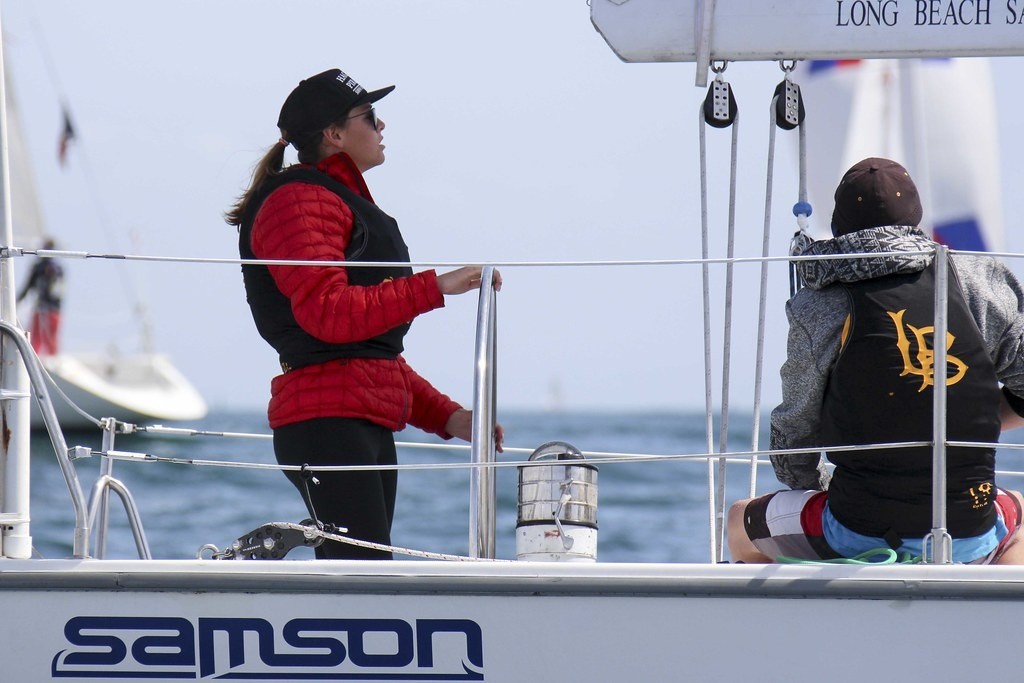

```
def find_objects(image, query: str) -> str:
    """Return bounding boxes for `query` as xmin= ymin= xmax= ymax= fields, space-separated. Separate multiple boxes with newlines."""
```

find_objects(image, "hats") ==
xmin=831 ymin=157 xmax=923 ymax=237
xmin=276 ymin=69 xmax=395 ymax=150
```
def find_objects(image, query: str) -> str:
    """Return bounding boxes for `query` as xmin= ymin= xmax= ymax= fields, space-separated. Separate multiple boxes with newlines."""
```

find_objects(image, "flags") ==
xmin=58 ymin=114 xmax=75 ymax=168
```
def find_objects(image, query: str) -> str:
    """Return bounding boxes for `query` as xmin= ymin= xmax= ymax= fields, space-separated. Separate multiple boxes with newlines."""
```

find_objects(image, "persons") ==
xmin=727 ymin=158 xmax=1024 ymax=565
xmin=222 ymin=69 xmax=504 ymax=561
xmin=14 ymin=238 xmax=65 ymax=354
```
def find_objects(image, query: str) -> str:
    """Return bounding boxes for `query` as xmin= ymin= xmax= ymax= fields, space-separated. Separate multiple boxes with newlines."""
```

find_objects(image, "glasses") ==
xmin=334 ymin=107 xmax=377 ymax=131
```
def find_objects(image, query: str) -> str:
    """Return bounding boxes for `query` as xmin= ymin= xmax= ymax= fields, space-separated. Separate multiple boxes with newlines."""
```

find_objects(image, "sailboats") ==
xmin=0 ymin=0 xmax=213 ymax=443
